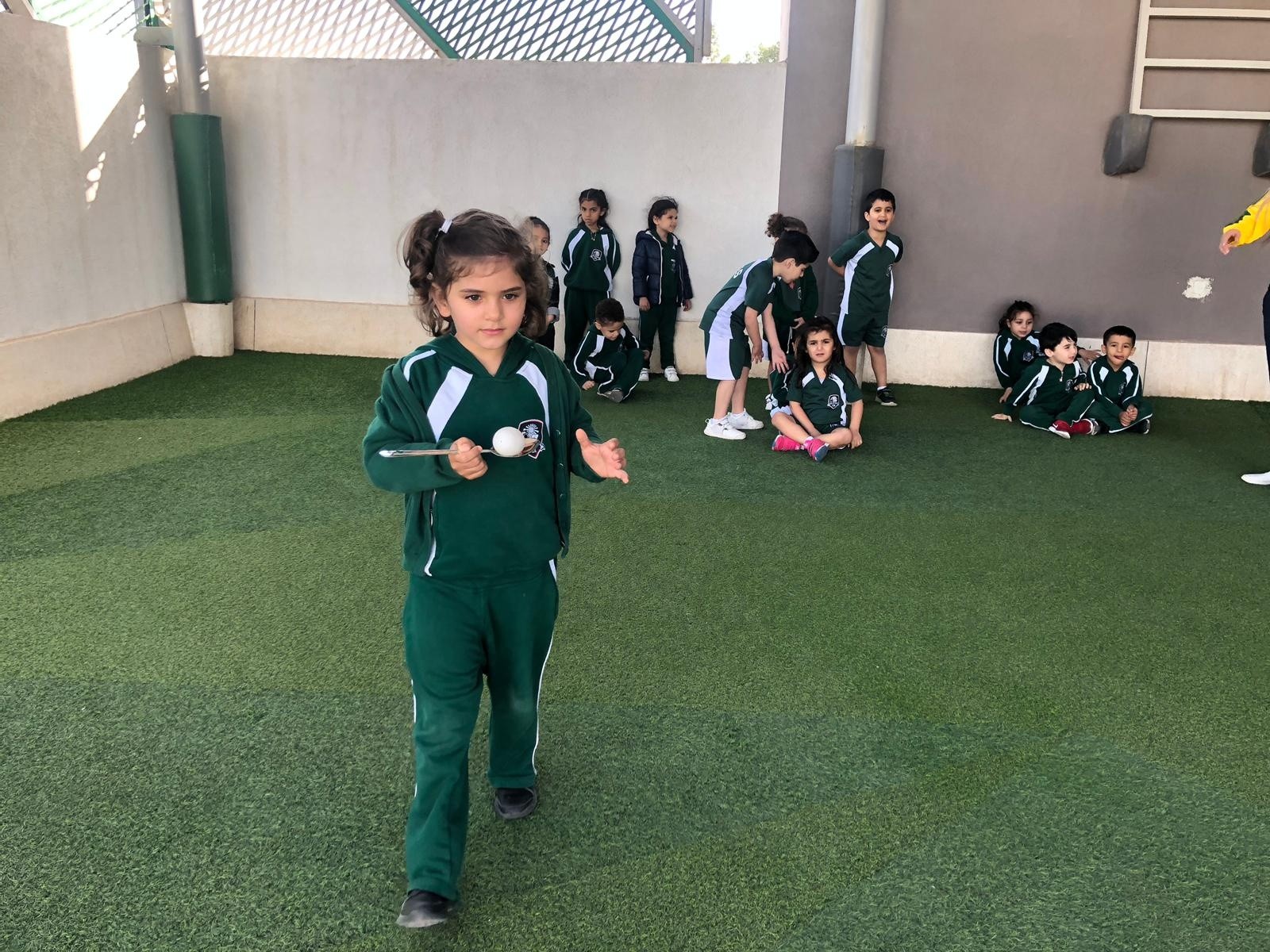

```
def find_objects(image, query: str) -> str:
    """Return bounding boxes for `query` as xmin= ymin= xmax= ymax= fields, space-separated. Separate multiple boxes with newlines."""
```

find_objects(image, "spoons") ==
xmin=379 ymin=438 xmax=541 ymax=458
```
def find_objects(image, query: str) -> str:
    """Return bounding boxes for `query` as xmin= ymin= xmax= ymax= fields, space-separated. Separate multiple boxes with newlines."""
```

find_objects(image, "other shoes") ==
xmin=596 ymin=385 xmax=624 ymax=403
xmin=1134 ymin=418 xmax=1151 ymax=435
xmin=1088 ymin=416 xmax=1104 ymax=435
xmin=1241 ymin=471 xmax=1270 ymax=485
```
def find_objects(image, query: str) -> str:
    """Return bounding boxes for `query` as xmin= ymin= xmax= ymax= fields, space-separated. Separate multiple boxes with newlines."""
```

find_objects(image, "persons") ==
xmin=574 ymin=298 xmax=643 ymax=402
xmin=828 ymin=187 xmax=905 ymax=406
xmin=362 ymin=209 xmax=627 ymax=927
xmin=632 ymin=196 xmax=694 ymax=381
xmin=766 ymin=214 xmax=819 ymax=410
xmin=991 ymin=300 xmax=1154 ymax=439
xmin=562 ymin=187 xmax=621 ymax=373
xmin=1219 ymin=187 xmax=1270 ymax=484
xmin=769 ymin=315 xmax=863 ymax=461
xmin=699 ymin=229 xmax=820 ymax=440
xmin=519 ymin=217 xmax=560 ymax=355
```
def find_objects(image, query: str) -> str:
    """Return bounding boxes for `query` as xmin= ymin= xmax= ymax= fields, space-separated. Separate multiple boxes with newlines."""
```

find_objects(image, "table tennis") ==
xmin=492 ymin=426 xmax=525 ymax=456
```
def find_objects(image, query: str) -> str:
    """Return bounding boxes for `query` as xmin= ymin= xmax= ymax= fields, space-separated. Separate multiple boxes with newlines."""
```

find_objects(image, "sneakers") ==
xmin=771 ymin=435 xmax=806 ymax=451
xmin=703 ymin=415 xmax=746 ymax=440
xmin=1048 ymin=420 xmax=1073 ymax=439
xmin=875 ymin=386 xmax=898 ymax=407
xmin=638 ymin=367 xmax=651 ymax=381
xmin=1073 ymin=418 xmax=1094 ymax=435
xmin=396 ymin=888 xmax=456 ymax=927
xmin=727 ymin=408 xmax=764 ymax=430
xmin=803 ymin=438 xmax=830 ymax=463
xmin=494 ymin=783 xmax=537 ymax=820
xmin=664 ymin=366 xmax=680 ymax=382
